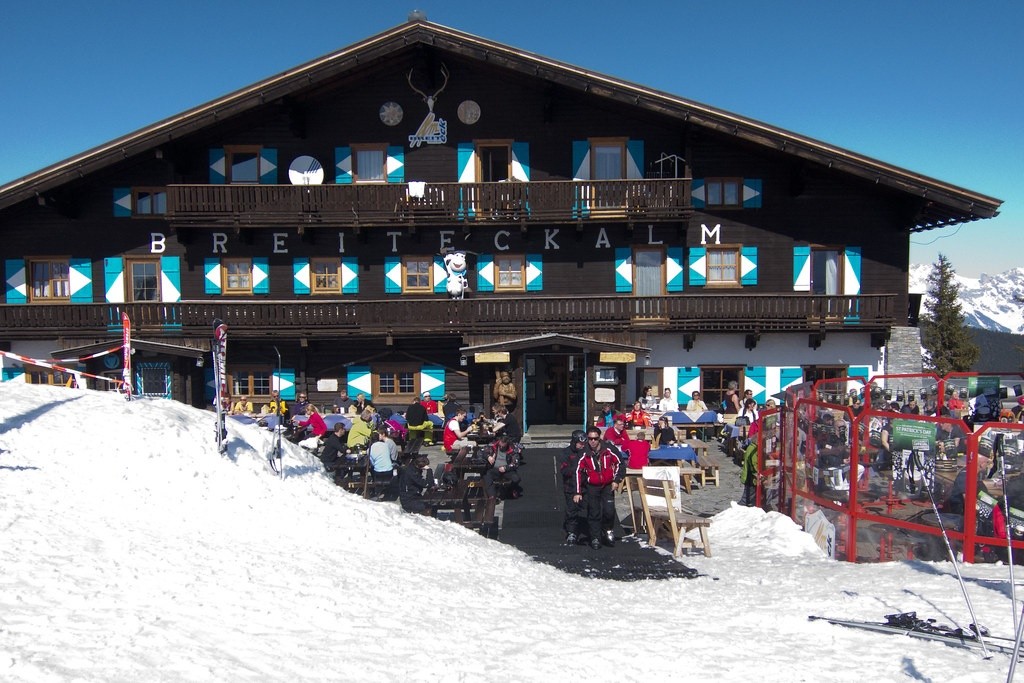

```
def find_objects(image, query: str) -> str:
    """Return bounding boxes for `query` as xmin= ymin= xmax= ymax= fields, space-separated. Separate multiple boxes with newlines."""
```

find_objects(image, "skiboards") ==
xmin=121 ymin=311 xmax=132 ymax=401
xmin=808 ymin=615 xmax=1024 ymax=657
xmin=213 ymin=318 xmax=227 ymax=442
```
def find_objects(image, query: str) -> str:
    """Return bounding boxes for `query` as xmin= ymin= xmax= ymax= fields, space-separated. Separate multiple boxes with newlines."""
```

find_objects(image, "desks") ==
xmin=621 ymin=444 xmax=697 ymax=462
xmin=933 ymin=454 xmax=966 ymax=485
xmin=373 ymin=413 xmax=444 ymax=429
xmin=424 ymin=476 xmax=474 ymax=525
xmin=332 ymin=447 xmax=367 ymax=471
xmin=467 ymin=429 xmax=495 ymax=444
xmin=228 ymin=413 xmax=279 ymax=430
xmin=452 ymin=445 xmax=493 ymax=478
xmin=293 ymin=414 xmax=384 ymax=431
xmin=662 ymin=412 xmax=718 ymax=428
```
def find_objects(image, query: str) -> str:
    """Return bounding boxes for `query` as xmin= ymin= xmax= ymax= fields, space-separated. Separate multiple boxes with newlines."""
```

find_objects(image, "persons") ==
xmin=483 ymin=436 xmax=521 ymax=499
xmin=444 ymin=408 xmax=476 ymax=451
xmin=725 ymin=381 xmax=740 ymax=414
xmin=560 ymin=430 xmax=589 ymax=544
xmin=221 ymin=392 xmax=235 ymax=414
xmin=737 ymin=389 xmax=1024 ymax=566
xmin=573 ymin=427 xmax=626 ymax=549
xmin=686 ymin=391 xmax=708 ymax=411
xmin=270 ymin=390 xmax=288 ymax=414
xmin=638 ymin=385 xmax=657 ymax=410
xmin=291 ymin=404 xmax=327 ymax=444
xmin=333 ymin=390 xmax=356 ymax=413
xmin=406 ymin=397 xmax=434 ymax=446
xmin=493 ymin=372 xmax=517 ymax=405
xmin=321 ymin=388 xmax=523 ymax=515
xmin=659 ymin=388 xmax=680 ymax=412
xmin=354 ymin=395 xmax=377 ymax=414
xmin=596 ymin=402 xmax=678 ymax=470
xmin=234 ymin=396 xmax=253 ymax=414
xmin=291 ymin=393 xmax=311 ymax=417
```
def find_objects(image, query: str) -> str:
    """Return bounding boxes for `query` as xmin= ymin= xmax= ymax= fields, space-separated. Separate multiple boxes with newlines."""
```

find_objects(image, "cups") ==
xmin=669 ymin=440 xmax=688 ymax=448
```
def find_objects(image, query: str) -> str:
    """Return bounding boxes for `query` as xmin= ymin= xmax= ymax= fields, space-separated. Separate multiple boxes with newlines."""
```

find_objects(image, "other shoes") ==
xmin=567 ymin=532 xmax=578 ymax=547
xmin=513 ymin=488 xmax=518 ymax=498
xmin=605 ymin=529 xmax=616 ymax=546
xmin=591 ymin=538 xmax=601 ymax=550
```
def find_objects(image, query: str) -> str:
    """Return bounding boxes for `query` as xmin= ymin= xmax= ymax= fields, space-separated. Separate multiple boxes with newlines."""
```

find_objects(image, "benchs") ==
xmin=637 ymin=436 xmax=719 ymax=559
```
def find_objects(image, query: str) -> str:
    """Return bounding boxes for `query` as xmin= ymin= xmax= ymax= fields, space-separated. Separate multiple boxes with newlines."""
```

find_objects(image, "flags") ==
xmin=121 ymin=312 xmax=132 ymax=392
xmin=212 ymin=318 xmax=232 ymax=408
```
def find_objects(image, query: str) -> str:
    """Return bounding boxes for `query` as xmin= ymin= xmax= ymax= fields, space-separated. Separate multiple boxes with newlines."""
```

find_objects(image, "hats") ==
xmin=978 ymin=447 xmax=990 ymax=458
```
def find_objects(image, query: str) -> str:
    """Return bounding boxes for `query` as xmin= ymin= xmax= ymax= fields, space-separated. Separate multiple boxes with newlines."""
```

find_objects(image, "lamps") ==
xmin=196 ymin=357 xmax=204 ymax=368
xmin=459 ymin=354 xmax=467 ymax=366
xmin=385 ymin=332 xmax=393 ymax=345
xmin=645 ymin=354 xmax=652 ymax=366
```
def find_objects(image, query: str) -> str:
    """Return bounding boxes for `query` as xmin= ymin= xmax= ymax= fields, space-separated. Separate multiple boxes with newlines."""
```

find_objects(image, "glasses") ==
xmin=766 ymin=404 xmax=770 ymax=406
xmin=588 ymin=437 xmax=599 ymax=440
xmin=299 ymin=396 xmax=306 ymax=398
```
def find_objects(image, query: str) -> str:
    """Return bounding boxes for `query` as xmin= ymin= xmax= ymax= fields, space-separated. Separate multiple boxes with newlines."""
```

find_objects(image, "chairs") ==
xmin=266 ymin=407 xmax=495 ymax=539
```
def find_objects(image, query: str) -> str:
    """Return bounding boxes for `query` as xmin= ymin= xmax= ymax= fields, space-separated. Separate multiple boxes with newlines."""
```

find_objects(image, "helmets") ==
xmin=572 ymin=429 xmax=588 ymax=444
